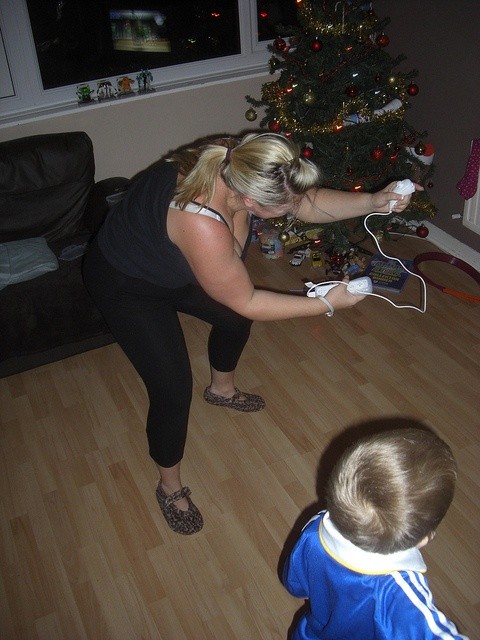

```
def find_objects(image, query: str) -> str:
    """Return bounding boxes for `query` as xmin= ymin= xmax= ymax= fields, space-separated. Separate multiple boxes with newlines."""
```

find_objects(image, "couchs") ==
xmin=1 ymin=131 xmax=131 ymax=377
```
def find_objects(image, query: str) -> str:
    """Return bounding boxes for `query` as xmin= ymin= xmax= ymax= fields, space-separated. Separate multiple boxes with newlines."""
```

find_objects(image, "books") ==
xmin=359 ymin=251 xmax=416 ymax=294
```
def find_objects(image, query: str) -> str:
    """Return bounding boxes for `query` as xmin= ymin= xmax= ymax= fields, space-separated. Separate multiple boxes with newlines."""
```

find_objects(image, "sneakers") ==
xmin=155 ymin=479 xmax=204 ymax=535
xmin=203 ymin=383 xmax=265 ymax=412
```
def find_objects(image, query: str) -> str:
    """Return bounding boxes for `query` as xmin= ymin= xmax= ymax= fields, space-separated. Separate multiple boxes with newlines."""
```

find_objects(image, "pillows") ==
xmin=0 ymin=237 xmax=58 ymax=292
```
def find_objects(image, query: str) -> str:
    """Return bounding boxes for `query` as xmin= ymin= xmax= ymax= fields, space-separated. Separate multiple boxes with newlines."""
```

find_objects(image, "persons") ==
xmin=281 ymin=425 xmax=474 ymax=639
xmin=78 ymin=128 xmax=427 ymax=536
